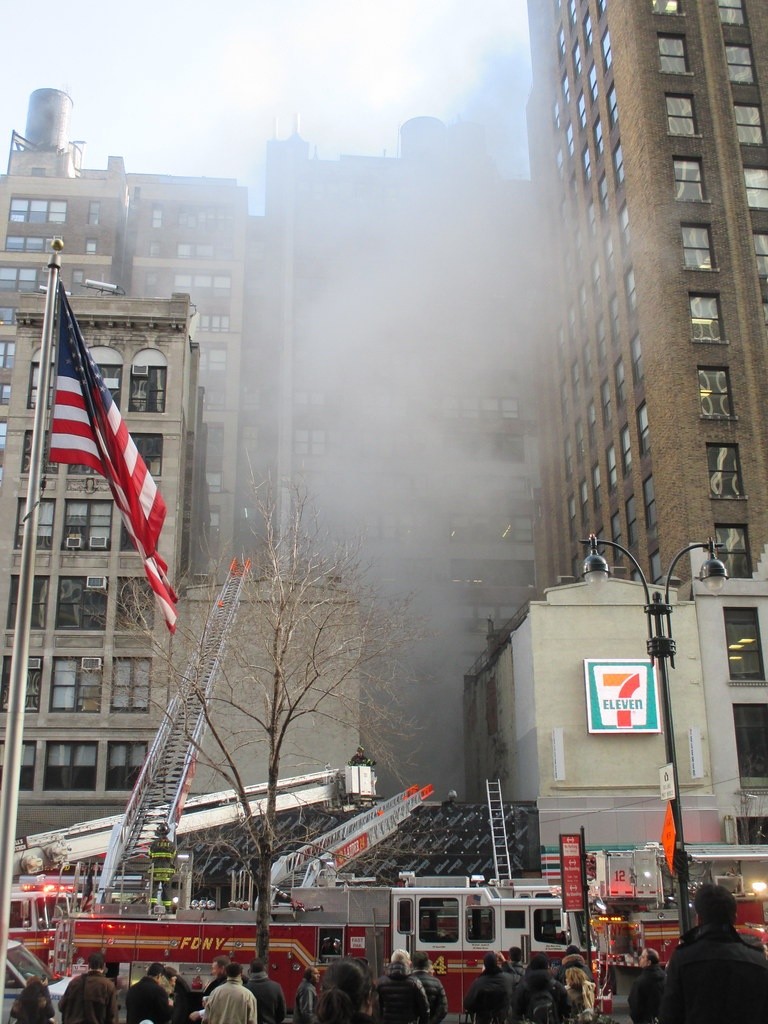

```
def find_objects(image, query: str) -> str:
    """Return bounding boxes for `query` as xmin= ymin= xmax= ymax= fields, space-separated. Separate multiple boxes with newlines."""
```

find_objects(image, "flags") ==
xmin=50 ymin=278 xmax=178 ymax=634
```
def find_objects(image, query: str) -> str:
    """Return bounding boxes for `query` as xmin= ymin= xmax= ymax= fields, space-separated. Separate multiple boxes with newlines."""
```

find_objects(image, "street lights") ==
xmin=583 ymin=532 xmax=730 ymax=936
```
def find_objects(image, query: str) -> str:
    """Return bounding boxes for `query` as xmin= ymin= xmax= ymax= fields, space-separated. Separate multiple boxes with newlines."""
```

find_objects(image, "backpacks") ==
xmin=523 ymin=979 xmax=560 ymax=1024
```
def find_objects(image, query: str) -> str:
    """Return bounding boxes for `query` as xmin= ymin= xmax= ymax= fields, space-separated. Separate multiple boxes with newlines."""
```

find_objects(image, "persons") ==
xmin=11 ymin=975 xmax=55 ymax=1024
xmin=662 ymin=884 xmax=768 ymax=1024
xmin=58 ymin=954 xmax=119 ymax=1024
xmin=126 ymin=946 xmax=594 ymax=1024
xmin=627 ymin=947 xmax=664 ymax=1024
xmin=349 ymin=747 xmax=376 ymax=767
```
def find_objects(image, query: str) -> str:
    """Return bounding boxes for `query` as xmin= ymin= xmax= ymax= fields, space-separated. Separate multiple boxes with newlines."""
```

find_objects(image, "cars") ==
xmin=4 ymin=939 xmax=71 ymax=1024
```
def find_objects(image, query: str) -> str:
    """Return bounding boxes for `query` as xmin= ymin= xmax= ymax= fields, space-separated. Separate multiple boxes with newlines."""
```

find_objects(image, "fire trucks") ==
xmin=583 ymin=844 xmax=768 ymax=959
xmin=68 ymin=556 xmax=613 ymax=1023
xmin=254 ymin=785 xmax=588 ymax=936
xmin=7 ymin=765 xmax=388 ymax=981
